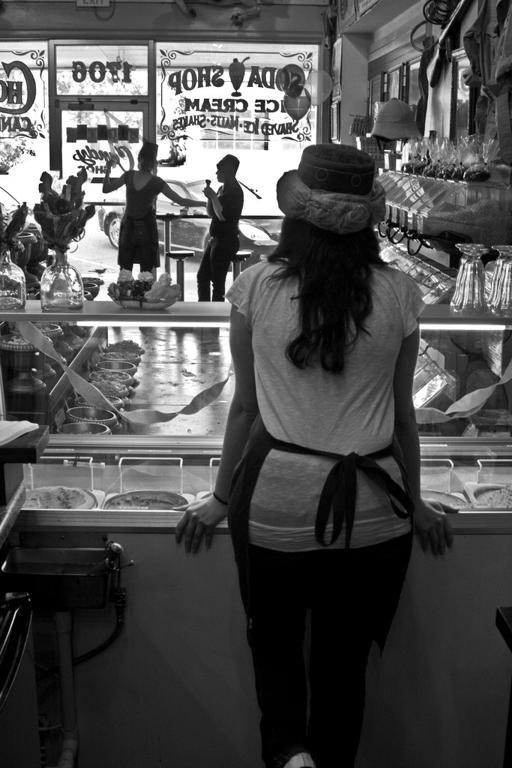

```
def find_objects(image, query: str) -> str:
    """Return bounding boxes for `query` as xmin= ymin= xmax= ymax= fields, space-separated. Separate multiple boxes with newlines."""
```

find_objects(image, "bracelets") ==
xmin=212 ymin=490 xmax=230 ymax=507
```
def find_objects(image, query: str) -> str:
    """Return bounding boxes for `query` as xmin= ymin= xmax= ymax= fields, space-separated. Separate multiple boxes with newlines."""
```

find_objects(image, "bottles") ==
xmin=0 ymin=248 xmax=29 ymax=313
xmin=37 ymin=246 xmax=88 ymax=312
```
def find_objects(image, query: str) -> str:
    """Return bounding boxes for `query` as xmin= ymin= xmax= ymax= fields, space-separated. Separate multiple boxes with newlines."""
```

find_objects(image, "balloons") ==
xmin=283 ymin=87 xmax=311 ymax=122
xmin=304 ymin=69 xmax=333 ymax=106
xmin=280 ymin=62 xmax=305 ymax=100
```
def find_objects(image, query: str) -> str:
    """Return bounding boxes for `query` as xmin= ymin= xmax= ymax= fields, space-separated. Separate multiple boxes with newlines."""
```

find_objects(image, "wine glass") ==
xmin=449 ymin=241 xmax=511 ymax=318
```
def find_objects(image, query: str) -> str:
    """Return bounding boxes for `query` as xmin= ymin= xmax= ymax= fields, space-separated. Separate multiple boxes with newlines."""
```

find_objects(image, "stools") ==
xmin=167 ymin=250 xmax=196 ymax=301
xmin=231 ymin=250 xmax=252 ymax=282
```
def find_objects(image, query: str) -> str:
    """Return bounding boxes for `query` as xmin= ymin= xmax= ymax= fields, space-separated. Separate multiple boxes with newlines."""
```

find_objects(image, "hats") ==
xmin=372 ymin=95 xmax=423 ymax=141
xmin=216 ymin=153 xmax=239 ymax=175
xmin=274 ymin=139 xmax=390 ymax=234
xmin=138 ymin=141 xmax=158 ymax=160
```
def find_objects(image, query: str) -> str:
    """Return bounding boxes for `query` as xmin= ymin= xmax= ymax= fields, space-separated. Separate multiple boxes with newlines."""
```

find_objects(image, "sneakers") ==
xmin=282 ymin=751 xmax=316 ymax=767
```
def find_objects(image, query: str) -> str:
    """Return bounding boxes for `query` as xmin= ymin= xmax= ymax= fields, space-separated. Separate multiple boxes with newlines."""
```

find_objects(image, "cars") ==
xmin=98 ymin=173 xmax=279 ymax=272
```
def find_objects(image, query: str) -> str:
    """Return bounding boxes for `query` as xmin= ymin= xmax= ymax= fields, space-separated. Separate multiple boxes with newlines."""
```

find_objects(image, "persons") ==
xmin=198 ymin=154 xmax=244 ymax=302
xmin=177 ymin=142 xmax=455 ymax=766
xmin=103 ymin=142 xmax=207 ymax=281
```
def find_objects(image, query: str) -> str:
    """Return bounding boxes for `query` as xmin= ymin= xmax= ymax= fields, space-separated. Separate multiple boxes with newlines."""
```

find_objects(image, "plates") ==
xmin=107 ymin=292 xmax=176 ymax=311
